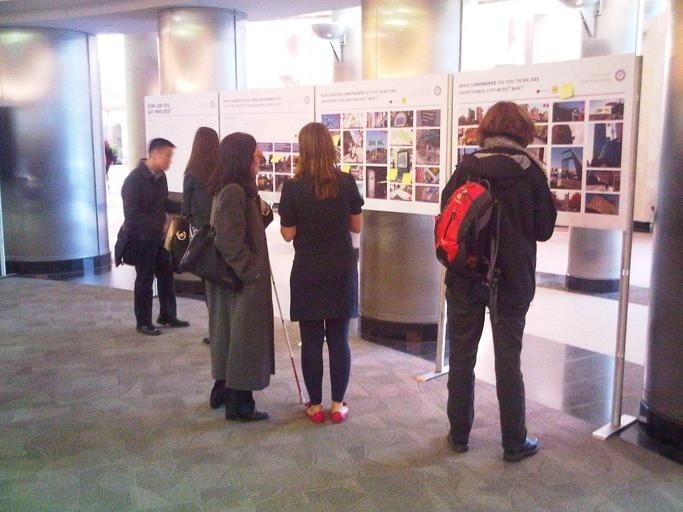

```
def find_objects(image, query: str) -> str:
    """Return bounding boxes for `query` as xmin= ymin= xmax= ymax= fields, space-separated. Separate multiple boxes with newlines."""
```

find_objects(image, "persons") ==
xmin=207 ymin=131 xmax=276 ymax=422
xmin=440 ymin=101 xmax=558 ymax=463
xmin=120 ymin=137 xmax=190 ymax=334
xmin=278 ymin=122 xmax=363 ymax=424
xmin=180 ymin=127 xmax=221 ymax=344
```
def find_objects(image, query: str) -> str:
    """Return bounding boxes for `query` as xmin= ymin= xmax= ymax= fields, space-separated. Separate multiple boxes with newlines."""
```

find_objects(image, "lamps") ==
xmin=313 ymin=21 xmax=346 ymax=60
xmin=561 ymin=0 xmax=599 ymax=37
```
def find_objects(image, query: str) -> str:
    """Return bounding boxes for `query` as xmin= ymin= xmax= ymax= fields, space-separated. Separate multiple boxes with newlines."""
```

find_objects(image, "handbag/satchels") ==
xmin=164 ymin=220 xmax=178 ymax=252
xmin=115 ymin=226 xmax=145 ymax=266
xmin=179 ymin=227 xmax=242 ymax=289
xmin=171 ymin=218 xmax=189 ymax=263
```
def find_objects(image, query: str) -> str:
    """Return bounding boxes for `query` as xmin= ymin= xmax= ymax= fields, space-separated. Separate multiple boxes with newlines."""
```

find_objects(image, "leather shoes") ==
xmin=136 ymin=324 xmax=161 ymax=335
xmin=157 ymin=318 xmax=189 ymax=327
xmin=304 ymin=401 xmax=325 ymax=422
xmin=331 ymin=401 xmax=349 ymax=423
xmin=447 ymin=432 xmax=469 ymax=452
xmin=226 ymin=408 xmax=267 ymax=420
xmin=503 ymin=438 xmax=538 ymax=462
xmin=210 ymin=388 xmax=225 ymax=409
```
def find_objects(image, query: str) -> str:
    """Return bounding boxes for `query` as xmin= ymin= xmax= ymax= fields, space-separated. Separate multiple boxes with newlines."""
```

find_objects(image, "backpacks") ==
xmin=434 ymin=171 xmax=502 ymax=282
